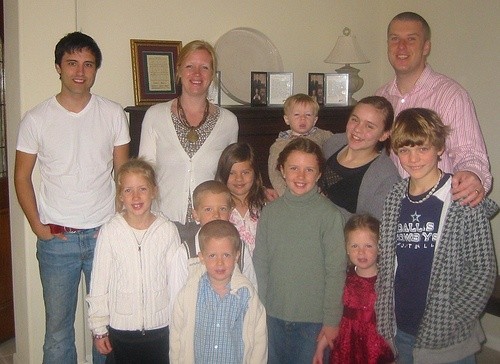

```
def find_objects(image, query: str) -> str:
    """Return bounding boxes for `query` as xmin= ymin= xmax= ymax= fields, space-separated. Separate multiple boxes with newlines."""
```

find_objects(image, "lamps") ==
xmin=323 ymin=25 xmax=370 ymax=105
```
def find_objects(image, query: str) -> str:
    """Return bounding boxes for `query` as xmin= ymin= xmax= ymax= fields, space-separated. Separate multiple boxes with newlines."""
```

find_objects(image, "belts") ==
xmin=49 ymin=224 xmax=79 ymax=234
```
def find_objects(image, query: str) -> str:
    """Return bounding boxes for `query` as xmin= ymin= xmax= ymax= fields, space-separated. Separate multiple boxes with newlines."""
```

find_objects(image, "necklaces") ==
xmin=404 ymin=168 xmax=442 ymax=204
xmin=176 ymin=95 xmax=210 ymax=143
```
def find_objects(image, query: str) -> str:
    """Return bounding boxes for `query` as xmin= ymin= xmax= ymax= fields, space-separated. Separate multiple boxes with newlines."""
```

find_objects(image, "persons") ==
xmin=375 ymin=11 xmax=493 ymax=207
xmin=268 ymin=92 xmax=334 ymax=196
xmin=372 ymin=104 xmax=500 ymax=364
xmin=311 ymin=75 xmax=323 ymax=103
xmin=312 ymin=214 xmax=397 ymax=364
xmin=167 ymin=142 xmax=267 ymax=364
xmin=317 ymin=95 xmax=401 ymax=225
xmin=87 ymin=158 xmax=183 ymax=364
xmin=13 ymin=32 xmax=131 ymax=364
xmin=140 ymin=39 xmax=280 ymax=238
xmin=252 ymin=74 xmax=267 ymax=106
xmin=254 ymin=139 xmax=345 ymax=364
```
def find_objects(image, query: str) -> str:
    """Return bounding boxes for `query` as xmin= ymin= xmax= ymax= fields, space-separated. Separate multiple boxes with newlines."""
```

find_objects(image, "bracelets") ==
xmin=92 ymin=332 xmax=109 ymax=340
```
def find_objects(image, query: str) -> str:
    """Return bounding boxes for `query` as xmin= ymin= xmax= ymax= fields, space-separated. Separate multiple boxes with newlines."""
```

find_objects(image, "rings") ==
xmin=475 ymin=190 xmax=480 ymax=195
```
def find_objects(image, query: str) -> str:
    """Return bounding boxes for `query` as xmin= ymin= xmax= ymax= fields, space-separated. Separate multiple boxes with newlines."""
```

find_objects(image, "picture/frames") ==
xmin=324 ymin=73 xmax=350 ymax=108
xmin=206 ymin=70 xmax=222 ymax=107
xmin=251 ymin=71 xmax=267 ymax=107
xmin=308 ymin=73 xmax=324 ymax=105
xmin=266 ymin=72 xmax=294 ymax=108
